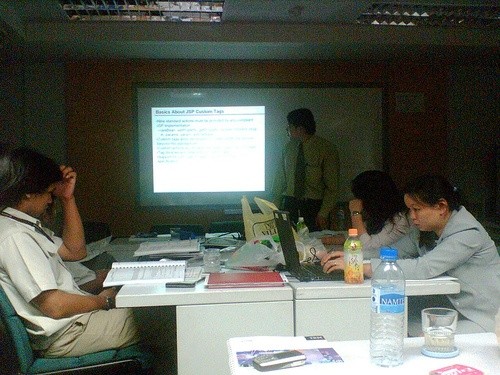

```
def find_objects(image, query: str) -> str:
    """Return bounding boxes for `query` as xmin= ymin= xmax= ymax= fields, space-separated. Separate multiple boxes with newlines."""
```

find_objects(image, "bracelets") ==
xmin=351 ymin=211 xmax=362 ymax=215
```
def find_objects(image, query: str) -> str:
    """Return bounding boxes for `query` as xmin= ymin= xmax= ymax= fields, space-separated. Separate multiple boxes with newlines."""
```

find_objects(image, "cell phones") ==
xmin=252 ymin=350 xmax=308 ymax=373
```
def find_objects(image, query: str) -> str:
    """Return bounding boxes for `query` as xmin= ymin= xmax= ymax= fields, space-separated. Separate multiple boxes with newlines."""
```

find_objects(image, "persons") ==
xmin=320 ymin=177 xmax=500 ymax=337
xmin=320 ymin=171 xmax=419 ymax=259
xmin=271 ymin=109 xmax=340 ymax=231
xmin=1 ymin=148 xmax=176 ymax=374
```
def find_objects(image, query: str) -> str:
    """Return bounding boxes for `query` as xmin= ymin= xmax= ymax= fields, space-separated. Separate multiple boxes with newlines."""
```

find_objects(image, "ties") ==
xmin=295 ymin=143 xmax=305 ymax=201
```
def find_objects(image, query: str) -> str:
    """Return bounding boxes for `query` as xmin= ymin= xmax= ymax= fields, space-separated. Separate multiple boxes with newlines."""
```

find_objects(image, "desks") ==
xmin=229 ymin=331 xmax=500 ymax=372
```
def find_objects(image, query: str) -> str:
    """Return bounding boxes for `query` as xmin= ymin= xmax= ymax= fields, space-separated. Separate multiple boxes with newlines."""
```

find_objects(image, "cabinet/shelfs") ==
xmin=286 ymin=230 xmax=460 ymax=338
xmin=118 ymin=240 xmax=293 ymax=372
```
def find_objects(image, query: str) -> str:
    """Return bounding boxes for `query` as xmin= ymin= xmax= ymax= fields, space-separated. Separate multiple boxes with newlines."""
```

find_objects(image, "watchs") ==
xmin=99 ymin=293 xmax=113 ymax=308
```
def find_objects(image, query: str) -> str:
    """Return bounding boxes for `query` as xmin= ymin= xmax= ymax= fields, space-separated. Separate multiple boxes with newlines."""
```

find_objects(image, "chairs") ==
xmin=0 ymin=289 xmax=153 ymax=374
xmin=63 ymin=222 xmax=111 ymax=244
xmin=90 ymin=239 xmax=97 ymax=243
xmin=154 ymin=221 xmax=209 ymax=240
xmin=213 ymin=216 xmax=260 ymax=239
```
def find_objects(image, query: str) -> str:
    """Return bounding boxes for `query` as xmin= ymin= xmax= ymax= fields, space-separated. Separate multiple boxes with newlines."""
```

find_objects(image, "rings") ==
xmin=335 ymin=260 xmax=336 ymax=265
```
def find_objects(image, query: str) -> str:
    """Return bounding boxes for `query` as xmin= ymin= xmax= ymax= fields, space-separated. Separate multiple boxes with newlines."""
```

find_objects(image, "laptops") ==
xmin=272 ymin=210 xmax=370 ymax=283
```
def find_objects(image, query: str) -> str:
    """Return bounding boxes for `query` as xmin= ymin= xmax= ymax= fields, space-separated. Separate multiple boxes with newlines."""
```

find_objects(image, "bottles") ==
xmin=343 ymin=229 xmax=364 ymax=284
xmin=369 ymin=247 xmax=405 ymax=356
xmin=296 ymin=217 xmax=309 ymax=237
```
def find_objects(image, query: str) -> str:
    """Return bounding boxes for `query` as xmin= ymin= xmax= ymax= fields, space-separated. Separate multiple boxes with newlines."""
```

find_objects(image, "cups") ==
xmin=420 ymin=307 xmax=459 ymax=357
xmin=202 ymin=249 xmax=222 ymax=273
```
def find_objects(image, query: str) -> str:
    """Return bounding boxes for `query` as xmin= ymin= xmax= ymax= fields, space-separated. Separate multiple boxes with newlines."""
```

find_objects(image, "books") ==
xmin=102 ymin=260 xmax=187 ymax=287
xmin=204 ymin=272 xmax=289 ymax=289
xmin=134 ymin=239 xmax=204 ymax=258
